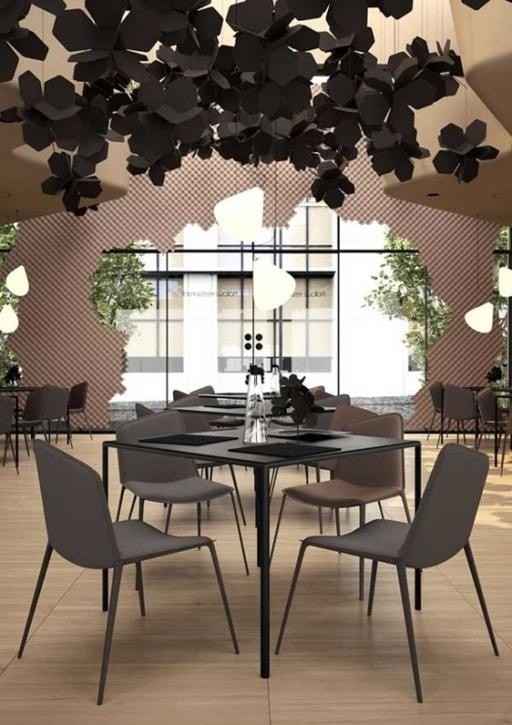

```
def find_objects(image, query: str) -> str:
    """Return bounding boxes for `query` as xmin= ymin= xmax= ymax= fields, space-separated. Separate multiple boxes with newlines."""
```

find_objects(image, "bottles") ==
xmin=242 ymin=362 xmax=269 ymax=445
xmin=269 ymin=364 xmax=282 ymax=396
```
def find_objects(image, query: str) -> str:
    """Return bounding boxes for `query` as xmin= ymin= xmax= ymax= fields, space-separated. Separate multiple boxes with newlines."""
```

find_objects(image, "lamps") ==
xmin=210 ymin=184 xmax=297 ymax=315
xmin=463 ymin=261 xmax=512 ymax=336
xmin=1 ymin=261 xmax=32 ymax=336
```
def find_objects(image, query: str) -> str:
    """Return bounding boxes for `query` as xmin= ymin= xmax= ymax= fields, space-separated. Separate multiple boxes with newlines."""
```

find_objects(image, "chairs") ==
xmin=103 ymin=385 xmax=423 ymax=679
xmin=19 ymin=439 xmax=240 ymax=705
xmin=1 ymin=381 xmax=92 ymax=475
xmin=427 ymin=381 xmax=512 ymax=477
xmin=275 ymin=442 xmax=499 ymax=703
